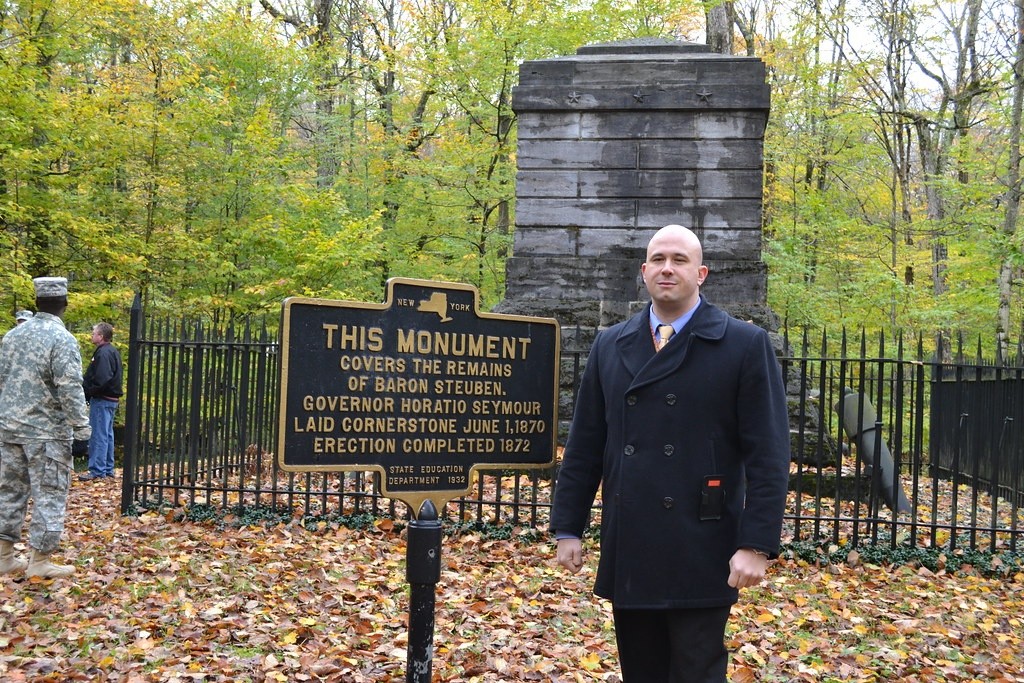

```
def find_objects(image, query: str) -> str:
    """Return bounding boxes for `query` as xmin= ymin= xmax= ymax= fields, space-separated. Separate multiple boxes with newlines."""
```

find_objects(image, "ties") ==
xmin=658 ymin=326 xmax=674 ymax=350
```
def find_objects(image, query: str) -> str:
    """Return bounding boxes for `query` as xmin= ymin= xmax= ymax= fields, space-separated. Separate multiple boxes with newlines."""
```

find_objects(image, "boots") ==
xmin=27 ymin=546 xmax=76 ymax=579
xmin=0 ymin=540 xmax=28 ymax=575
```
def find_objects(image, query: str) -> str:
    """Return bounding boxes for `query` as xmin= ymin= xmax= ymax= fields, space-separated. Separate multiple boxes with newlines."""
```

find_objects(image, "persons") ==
xmin=1 ymin=276 xmax=93 ymax=577
xmin=547 ymin=225 xmax=790 ymax=682
xmin=15 ymin=309 xmax=33 ymax=326
xmin=78 ymin=321 xmax=124 ymax=481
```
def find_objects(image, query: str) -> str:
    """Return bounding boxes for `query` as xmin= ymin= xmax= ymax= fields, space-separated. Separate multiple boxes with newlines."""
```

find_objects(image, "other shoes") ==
xmin=79 ymin=473 xmax=105 ymax=481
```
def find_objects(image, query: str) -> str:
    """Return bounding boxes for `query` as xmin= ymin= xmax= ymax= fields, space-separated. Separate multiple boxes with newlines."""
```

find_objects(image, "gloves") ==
xmin=71 ymin=439 xmax=89 ymax=458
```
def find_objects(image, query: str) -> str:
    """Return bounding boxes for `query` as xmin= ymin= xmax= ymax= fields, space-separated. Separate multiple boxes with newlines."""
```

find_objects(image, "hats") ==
xmin=15 ymin=310 xmax=33 ymax=321
xmin=33 ymin=277 xmax=68 ymax=297
